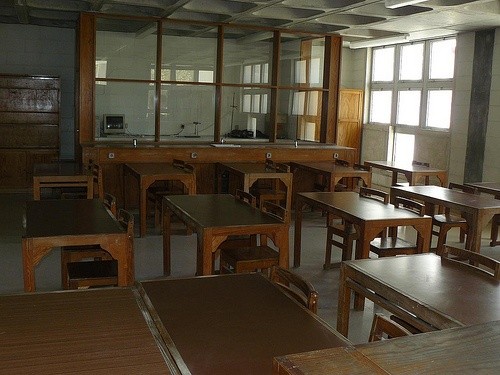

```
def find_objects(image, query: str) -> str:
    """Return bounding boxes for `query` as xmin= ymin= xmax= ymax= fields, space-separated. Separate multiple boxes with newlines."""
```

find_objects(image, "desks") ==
xmin=1 ymin=160 xmax=499 ymax=375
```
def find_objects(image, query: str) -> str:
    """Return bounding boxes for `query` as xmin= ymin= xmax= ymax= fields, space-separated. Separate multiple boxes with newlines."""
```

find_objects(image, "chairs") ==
xmin=56 ymin=158 xmax=500 ymax=341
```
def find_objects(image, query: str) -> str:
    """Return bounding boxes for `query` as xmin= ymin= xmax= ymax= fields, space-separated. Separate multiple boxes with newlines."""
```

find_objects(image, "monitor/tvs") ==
xmin=103 ymin=114 xmax=126 ymax=135
